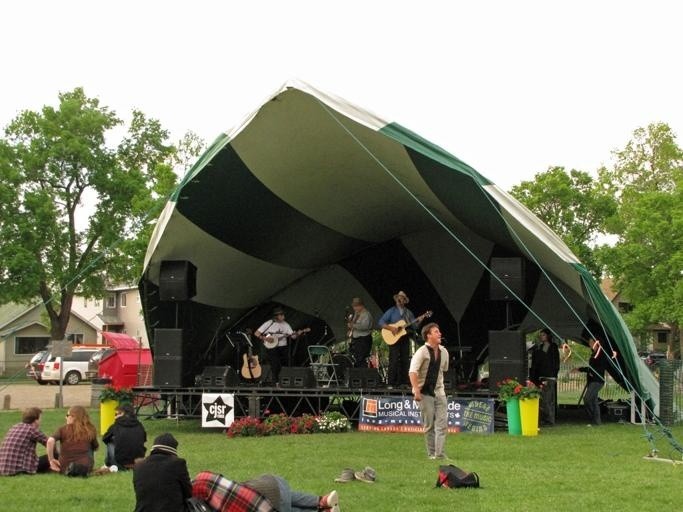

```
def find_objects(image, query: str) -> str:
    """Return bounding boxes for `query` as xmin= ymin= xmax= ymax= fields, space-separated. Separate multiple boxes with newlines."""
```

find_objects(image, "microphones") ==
xmin=324 ymin=325 xmax=328 ymax=335
xmin=220 ymin=315 xmax=231 ymax=320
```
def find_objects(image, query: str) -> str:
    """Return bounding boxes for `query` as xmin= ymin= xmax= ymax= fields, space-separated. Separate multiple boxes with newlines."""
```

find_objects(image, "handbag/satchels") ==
xmin=187 ymin=497 xmax=211 ymax=512
xmin=64 ymin=463 xmax=88 ymax=477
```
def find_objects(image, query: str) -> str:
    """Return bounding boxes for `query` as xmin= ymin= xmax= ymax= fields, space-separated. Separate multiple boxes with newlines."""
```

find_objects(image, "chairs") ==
xmin=308 ymin=345 xmax=339 ymax=386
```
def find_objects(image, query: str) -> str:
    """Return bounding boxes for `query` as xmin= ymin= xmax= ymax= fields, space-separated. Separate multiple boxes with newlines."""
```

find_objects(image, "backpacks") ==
xmin=436 ymin=465 xmax=479 ymax=488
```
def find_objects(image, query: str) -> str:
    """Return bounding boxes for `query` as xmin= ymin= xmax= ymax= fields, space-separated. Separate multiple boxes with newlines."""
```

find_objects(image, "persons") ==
xmin=132 ymin=433 xmax=191 ymax=512
xmin=379 ymin=291 xmax=425 ymax=391
xmin=409 ymin=324 xmax=450 ymax=461
xmin=47 ymin=406 xmax=99 ymax=476
xmin=188 ymin=469 xmax=340 ymax=512
xmin=347 ymin=297 xmax=374 ymax=386
xmin=529 ymin=329 xmax=560 ymax=428
xmin=569 ymin=339 xmax=605 ymax=426
xmin=253 ymin=308 xmax=305 ymax=383
xmin=0 ymin=408 xmax=59 ymax=477
xmin=102 ymin=402 xmax=148 ymax=471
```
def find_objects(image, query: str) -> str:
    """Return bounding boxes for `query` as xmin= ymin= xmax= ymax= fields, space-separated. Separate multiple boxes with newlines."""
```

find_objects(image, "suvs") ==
xmin=25 ymin=345 xmax=100 ymax=385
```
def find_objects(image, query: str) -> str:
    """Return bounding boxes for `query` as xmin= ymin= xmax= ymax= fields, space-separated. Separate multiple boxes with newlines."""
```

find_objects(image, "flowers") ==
xmin=515 ymin=381 xmax=543 ymax=401
xmin=98 ymin=384 xmax=134 ymax=406
xmin=496 ymin=378 xmax=521 ymax=401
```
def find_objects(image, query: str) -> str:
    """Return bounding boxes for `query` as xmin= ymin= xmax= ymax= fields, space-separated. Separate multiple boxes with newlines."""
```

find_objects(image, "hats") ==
xmin=393 ymin=290 xmax=410 ymax=304
xmin=153 ymin=431 xmax=180 ymax=449
xmin=350 ymin=297 xmax=364 ymax=306
xmin=114 ymin=404 xmax=134 ymax=413
xmin=271 ymin=308 xmax=287 ymax=316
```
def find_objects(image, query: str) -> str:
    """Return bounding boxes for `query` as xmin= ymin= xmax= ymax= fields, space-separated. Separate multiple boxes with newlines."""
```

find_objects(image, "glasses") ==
xmin=115 ymin=412 xmax=121 ymax=416
xmin=67 ymin=414 xmax=71 ymax=417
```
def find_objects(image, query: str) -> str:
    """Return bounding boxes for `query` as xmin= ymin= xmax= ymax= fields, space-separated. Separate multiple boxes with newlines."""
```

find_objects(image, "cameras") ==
xmin=579 ymin=367 xmax=589 ymax=373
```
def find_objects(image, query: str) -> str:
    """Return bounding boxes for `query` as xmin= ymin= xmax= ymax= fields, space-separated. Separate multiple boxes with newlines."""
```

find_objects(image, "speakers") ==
xmin=443 ymin=368 xmax=456 ymax=390
xmin=488 ymin=330 xmax=526 ymax=393
xmin=489 ymin=258 xmax=525 ymax=301
xmin=201 ymin=366 xmax=241 ymax=387
xmin=153 ymin=328 xmax=183 ymax=387
xmin=346 ymin=368 xmax=383 ymax=388
xmin=278 ymin=367 xmax=317 ymax=388
xmin=159 ymin=260 xmax=198 ymax=301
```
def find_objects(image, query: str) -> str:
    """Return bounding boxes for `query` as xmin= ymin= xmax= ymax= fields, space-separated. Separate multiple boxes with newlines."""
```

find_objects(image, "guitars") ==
xmin=345 ymin=314 xmax=355 ymax=344
xmin=382 ymin=311 xmax=432 ymax=345
xmin=241 ymin=329 xmax=263 ymax=379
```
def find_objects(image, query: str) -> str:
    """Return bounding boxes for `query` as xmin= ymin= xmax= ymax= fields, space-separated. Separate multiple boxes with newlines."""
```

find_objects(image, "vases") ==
xmin=100 ymin=400 xmax=118 ymax=435
xmin=507 ymin=399 xmax=522 ymax=435
xmin=519 ymin=398 xmax=539 ymax=436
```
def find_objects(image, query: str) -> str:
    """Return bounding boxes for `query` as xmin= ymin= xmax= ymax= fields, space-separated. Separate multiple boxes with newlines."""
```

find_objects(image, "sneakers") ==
xmin=354 ymin=466 xmax=376 ymax=483
xmin=319 ymin=490 xmax=338 ymax=508
xmin=386 ymin=384 xmax=393 ymax=390
xmin=320 ymin=506 xmax=340 ymax=512
xmin=542 ymin=421 xmax=553 ymax=427
xmin=90 ymin=465 xmax=110 ymax=474
xmin=400 ymin=384 xmax=412 ymax=390
xmin=333 ymin=469 xmax=354 ymax=484
